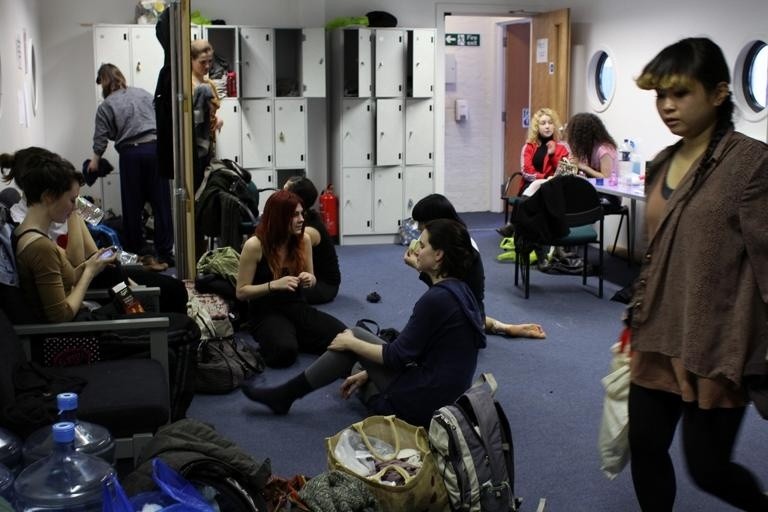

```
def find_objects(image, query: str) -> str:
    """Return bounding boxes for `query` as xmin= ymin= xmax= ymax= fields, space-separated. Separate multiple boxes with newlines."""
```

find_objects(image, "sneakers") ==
xmin=496 ymin=226 xmax=513 ymax=238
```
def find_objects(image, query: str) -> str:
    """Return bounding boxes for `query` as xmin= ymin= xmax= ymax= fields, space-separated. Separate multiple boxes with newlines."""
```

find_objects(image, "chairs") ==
xmin=0 ymin=313 xmax=172 ymax=468
xmin=513 ymin=174 xmax=605 ymax=300
xmin=0 ymin=207 xmax=160 ymax=333
xmin=500 ymin=160 xmax=530 ymax=254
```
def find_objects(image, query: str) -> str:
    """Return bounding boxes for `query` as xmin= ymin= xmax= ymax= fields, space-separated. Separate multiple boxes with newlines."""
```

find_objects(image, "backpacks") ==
xmin=431 ymin=385 xmax=522 ymax=512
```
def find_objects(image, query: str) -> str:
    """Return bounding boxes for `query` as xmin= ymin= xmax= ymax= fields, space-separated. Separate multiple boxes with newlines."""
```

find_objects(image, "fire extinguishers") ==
xmin=317 ymin=182 xmax=338 ymax=238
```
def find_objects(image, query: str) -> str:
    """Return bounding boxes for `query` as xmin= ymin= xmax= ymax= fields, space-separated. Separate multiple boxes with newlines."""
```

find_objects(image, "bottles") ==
xmin=618 ymin=139 xmax=632 ymax=177
xmin=75 ymin=193 xmax=104 ymax=226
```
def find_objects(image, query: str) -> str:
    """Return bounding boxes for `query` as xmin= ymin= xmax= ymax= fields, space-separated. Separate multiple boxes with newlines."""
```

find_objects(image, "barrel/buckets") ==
xmin=0 ymin=457 xmax=13 ymax=495
xmin=14 ymin=422 xmax=117 ymax=507
xmin=0 ymin=427 xmax=25 ymax=475
xmin=23 ymin=390 xmax=116 ymax=472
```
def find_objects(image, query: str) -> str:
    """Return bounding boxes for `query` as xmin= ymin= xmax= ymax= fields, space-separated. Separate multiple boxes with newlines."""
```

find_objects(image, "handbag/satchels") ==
xmin=600 ymin=325 xmax=633 ymax=480
xmin=324 ymin=412 xmax=451 ymax=512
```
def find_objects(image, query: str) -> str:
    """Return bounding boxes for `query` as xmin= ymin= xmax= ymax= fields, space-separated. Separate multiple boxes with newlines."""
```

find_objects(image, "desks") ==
xmin=585 ymin=158 xmax=647 ymax=209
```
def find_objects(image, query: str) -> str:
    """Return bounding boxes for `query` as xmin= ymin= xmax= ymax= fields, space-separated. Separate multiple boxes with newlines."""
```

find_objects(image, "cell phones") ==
xmin=98 ymin=246 xmax=117 ymax=262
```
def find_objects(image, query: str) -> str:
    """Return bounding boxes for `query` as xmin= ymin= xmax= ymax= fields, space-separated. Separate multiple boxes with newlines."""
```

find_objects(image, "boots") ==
xmin=536 ymin=245 xmax=549 ymax=270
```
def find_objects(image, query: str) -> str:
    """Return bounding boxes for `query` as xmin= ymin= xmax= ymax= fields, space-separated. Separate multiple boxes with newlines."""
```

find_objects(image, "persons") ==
xmin=9 ymin=154 xmax=202 ymax=424
xmin=518 ymin=108 xmax=571 ymax=199
xmin=403 ymin=193 xmax=547 ymax=341
xmin=0 ymin=147 xmax=189 ymax=315
xmin=235 ymin=191 xmax=348 ymax=371
xmin=238 ymin=218 xmax=488 ymax=430
xmin=284 ymin=175 xmax=341 ymax=305
xmin=191 ymin=39 xmax=224 ymax=189
xmin=495 ymin=112 xmax=619 ymax=271
xmin=621 ymin=34 xmax=768 ymax=512
xmin=88 ymin=64 xmax=175 ymax=266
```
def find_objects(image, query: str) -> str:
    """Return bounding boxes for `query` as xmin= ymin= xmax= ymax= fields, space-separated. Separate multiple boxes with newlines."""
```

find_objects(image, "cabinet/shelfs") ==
xmin=90 ymin=23 xmax=202 ymax=218
xmin=198 ymin=26 xmax=327 ymax=216
xmin=331 ymin=25 xmax=436 ymax=247
